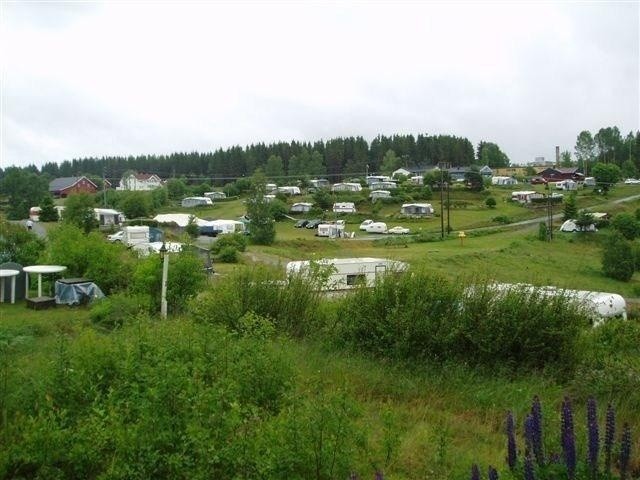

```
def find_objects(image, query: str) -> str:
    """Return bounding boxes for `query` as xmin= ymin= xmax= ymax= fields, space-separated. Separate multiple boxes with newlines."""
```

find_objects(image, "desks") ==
xmin=23 ymin=265 xmax=68 ymax=298
xmin=0 ymin=269 xmax=20 ymax=304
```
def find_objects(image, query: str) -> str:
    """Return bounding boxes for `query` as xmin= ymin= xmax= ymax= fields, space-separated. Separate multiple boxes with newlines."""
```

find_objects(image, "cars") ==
xmin=389 ymin=227 xmax=409 ymax=234
xmin=532 ymin=178 xmax=547 ymax=185
xmin=360 ymin=220 xmax=373 ymax=230
xmin=625 ymin=179 xmax=640 ymax=184
xmin=107 ymin=231 xmax=125 ymax=243
xmin=294 ymin=220 xmax=322 ymax=229
